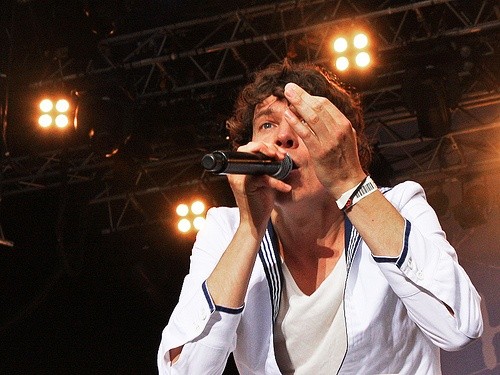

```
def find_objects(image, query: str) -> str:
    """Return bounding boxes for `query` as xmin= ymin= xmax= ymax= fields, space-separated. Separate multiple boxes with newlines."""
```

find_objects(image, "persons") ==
xmin=156 ymin=61 xmax=484 ymax=375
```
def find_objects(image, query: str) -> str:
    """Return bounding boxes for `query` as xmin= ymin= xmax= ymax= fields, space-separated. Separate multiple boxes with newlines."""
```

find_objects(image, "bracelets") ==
xmin=335 ymin=176 xmax=377 ymax=212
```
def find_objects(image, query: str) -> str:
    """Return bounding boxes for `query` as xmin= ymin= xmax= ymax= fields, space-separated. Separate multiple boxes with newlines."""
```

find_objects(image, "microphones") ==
xmin=201 ymin=150 xmax=293 ymax=181
